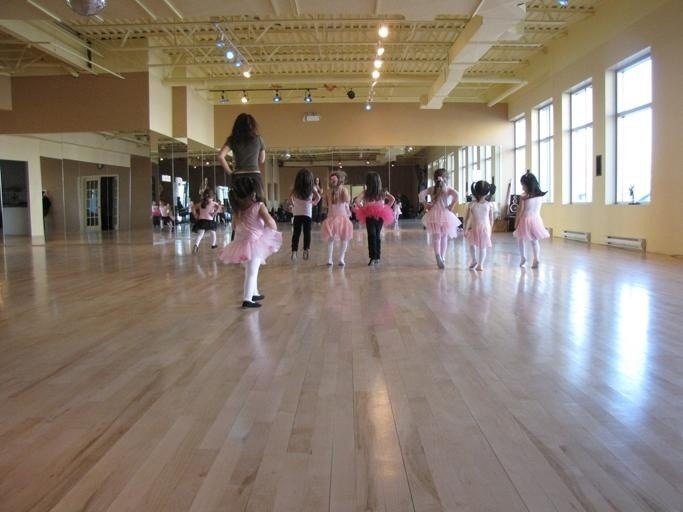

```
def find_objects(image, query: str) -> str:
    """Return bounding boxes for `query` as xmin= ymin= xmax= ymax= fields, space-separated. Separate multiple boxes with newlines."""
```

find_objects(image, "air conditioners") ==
xmin=604 ymin=235 xmax=646 ymax=251
xmin=562 ymin=230 xmax=592 ymax=242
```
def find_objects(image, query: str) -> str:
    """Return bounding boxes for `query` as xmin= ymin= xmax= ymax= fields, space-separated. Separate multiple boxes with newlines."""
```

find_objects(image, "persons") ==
xmin=351 ymin=171 xmax=396 ymax=266
xmin=192 ymin=189 xmax=219 ymax=255
xmin=418 ymin=168 xmax=462 ymax=269
xmin=313 ymin=177 xmax=323 ymax=224
xmin=394 ymin=198 xmax=402 ymax=221
xmin=288 ymin=168 xmax=321 ymax=261
xmin=218 ymin=112 xmax=266 ymax=241
xmin=513 ymin=170 xmax=550 ymax=269
xmin=321 ymin=171 xmax=353 ymax=266
xmin=151 ymin=197 xmax=175 ymax=235
xmin=220 ymin=174 xmax=282 ymax=309
xmin=462 ymin=180 xmax=496 ymax=271
xmin=199 ymin=177 xmax=209 ymax=197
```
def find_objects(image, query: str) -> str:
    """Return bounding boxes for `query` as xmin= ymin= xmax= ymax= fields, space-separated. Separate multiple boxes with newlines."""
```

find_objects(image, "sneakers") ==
xmin=291 ymin=249 xmax=297 ymax=261
xmin=242 ymin=301 xmax=262 ymax=308
xmin=368 ymin=259 xmax=375 ymax=266
xmin=191 ymin=245 xmax=199 ymax=256
xmin=303 ymin=250 xmax=308 ymax=260
xmin=376 ymin=259 xmax=380 ymax=263
xmin=252 ymin=295 xmax=265 ymax=300
xmin=211 ymin=245 xmax=217 ymax=249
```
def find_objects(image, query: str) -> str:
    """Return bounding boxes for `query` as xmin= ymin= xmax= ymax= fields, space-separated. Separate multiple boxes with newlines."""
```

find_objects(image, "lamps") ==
xmin=217 ymin=91 xmax=229 ymax=105
xmin=215 ymin=31 xmax=225 ymax=48
xmin=303 ymin=89 xmax=312 ymax=102
xmin=272 ymin=90 xmax=282 ymax=103
xmin=238 ymin=91 xmax=253 ymax=103
xmin=242 ymin=65 xmax=258 ymax=78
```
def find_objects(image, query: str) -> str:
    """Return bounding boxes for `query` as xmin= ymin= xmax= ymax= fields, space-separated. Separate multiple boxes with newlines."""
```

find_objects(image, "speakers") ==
xmin=509 ymin=195 xmax=521 ymax=231
